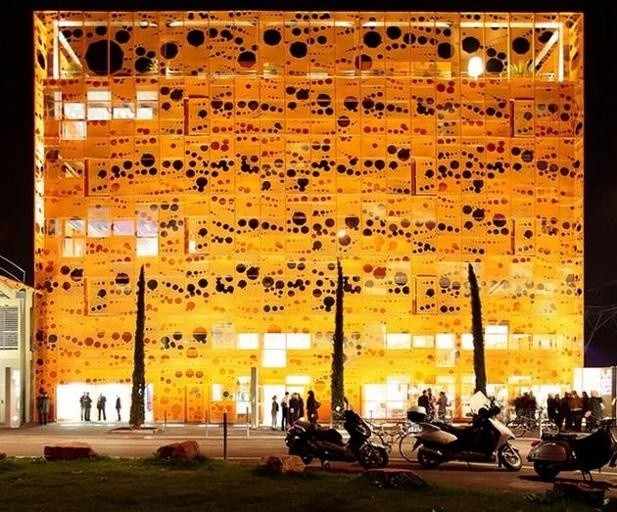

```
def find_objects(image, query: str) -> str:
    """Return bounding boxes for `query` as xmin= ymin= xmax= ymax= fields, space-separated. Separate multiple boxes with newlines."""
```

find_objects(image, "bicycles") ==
xmin=502 ymin=405 xmax=560 ymax=440
xmin=351 ymin=414 xmax=426 ymax=463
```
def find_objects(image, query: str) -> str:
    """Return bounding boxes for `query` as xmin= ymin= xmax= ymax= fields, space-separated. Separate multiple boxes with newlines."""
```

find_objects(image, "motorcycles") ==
xmin=283 ymin=397 xmax=391 ymax=472
xmin=405 ymin=395 xmax=522 ymax=474
xmin=526 ymin=398 xmax=617 ymax=486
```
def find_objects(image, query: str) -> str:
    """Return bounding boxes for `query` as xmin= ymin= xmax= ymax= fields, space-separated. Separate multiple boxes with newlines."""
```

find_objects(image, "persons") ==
xmin=418 ymin=388 xmax=447 ymax=418
xmin=37 ymin=392 xmax=50 ymax=425
xmin=116 ymin=395 xmax=121 ymax=421
xmin=513 ymin=390 xmax=537 ymax=426
xmin=80 ymin=392 xmax=92 ymax=421
xmin=307 ymin=391 xmax=321 ymax=426
xmin=272 ymin=392 xmax=303 ymax=431
xmin=97 ymin=393 xmax=106 ymax=421
xmin=547 ymin=391 xmax=603 ymax=431
